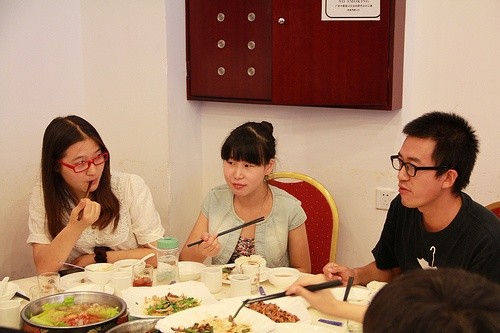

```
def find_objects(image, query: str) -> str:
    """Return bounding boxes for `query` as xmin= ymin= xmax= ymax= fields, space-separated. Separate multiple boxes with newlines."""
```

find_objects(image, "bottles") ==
xmin=157 ymin=237 xmax=180 ymax=284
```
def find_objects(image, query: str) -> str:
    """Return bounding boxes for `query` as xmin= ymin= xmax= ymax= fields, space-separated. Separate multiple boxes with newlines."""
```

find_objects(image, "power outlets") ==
xmin=375 ymin=188 xmax=398 ymax=209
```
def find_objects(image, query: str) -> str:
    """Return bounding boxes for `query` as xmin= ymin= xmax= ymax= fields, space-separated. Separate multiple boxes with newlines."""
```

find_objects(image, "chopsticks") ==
xmin=77 ymin=181 xmax=93 ymax=221
xmin=187 ymin=216 xmax=265 ymax=247
xmin=248 ymin=279 xmax=343 ymax=304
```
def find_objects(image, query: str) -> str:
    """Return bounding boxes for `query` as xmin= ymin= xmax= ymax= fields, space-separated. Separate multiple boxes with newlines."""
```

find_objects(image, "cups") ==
xmin=109 ymin=273 xmax=132 ymax=294
xmin=39 ymin=270 xmax=61 ymax=295
xmin=228 ymin=273 xmax=251 ymax=298
xmin=132 ymin=264 xmax=153 ymax=287
xmin=0 ymin=301 xmax=21 ymax=329
xmin=240 ymin=262 xmax=261 ymax=294
xmin=200 ymin=268 xmax=223 ymax=293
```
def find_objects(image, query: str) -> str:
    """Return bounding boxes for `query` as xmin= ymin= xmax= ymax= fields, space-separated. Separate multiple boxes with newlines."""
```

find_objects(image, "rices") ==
xmin=236 ymin=254 xmax=265 ymax=265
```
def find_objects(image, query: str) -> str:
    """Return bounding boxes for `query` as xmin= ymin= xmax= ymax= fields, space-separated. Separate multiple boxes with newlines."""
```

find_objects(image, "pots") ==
xmin=21 ymin=291 xmax=127 ymax=333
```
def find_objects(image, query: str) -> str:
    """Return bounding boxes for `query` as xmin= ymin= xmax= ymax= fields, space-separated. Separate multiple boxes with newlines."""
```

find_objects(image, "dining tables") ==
xmin=0 ymin=266 xmax=374 ymax=333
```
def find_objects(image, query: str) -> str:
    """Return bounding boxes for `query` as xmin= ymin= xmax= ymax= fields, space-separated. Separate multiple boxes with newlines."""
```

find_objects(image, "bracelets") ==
xmin=94 ymin=247 xmax=107 ymax=263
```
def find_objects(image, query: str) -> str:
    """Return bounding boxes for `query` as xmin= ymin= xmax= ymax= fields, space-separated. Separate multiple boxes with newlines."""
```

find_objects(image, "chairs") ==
xmin=267 ymin=172 xmax=339 ymax=274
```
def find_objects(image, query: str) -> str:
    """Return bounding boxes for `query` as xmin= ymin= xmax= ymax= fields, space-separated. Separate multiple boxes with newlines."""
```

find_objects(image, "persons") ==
xmin=179 ymin=121 xmax=311 ymax=274
xmin=322 ymin=112 xmax=500 ymax=288
xmin=26 ymin=115 xmax=165 ymax=275
xmin=285 ymin=267 xmax=500 ymax=333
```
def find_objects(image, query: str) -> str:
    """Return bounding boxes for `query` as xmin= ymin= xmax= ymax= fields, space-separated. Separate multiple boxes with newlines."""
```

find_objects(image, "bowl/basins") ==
xmin=84 ymin=263 xmax=115 ymax=284
xmin=331 ymin=285 xmax=372 ymax=306
xmin=0 ymin=281 xmax=17 ymax=301
xmin=106 ymin=318 xmax=166 ymax=333
xmin=267 ymin=267 xmax=300 ymax=289
xmin=114 ymin=259 xmax=146 ymax=274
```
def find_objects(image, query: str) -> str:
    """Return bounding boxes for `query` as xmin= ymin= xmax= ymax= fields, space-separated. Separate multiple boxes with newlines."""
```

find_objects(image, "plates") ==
xmin=123 ymin=282 xmax=329 ymax=333
xmin=58 ymin=271 xmax=121 ymax=294
xmin=177 ymin=261 xmax=206 ymax=281
xmin=218 ymin=263 xmax=272 ymax=284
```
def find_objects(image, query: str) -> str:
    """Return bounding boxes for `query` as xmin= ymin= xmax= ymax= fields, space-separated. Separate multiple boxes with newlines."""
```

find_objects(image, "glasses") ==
xmin=56 ymin=152 xmax=109 ymax=173
xmin=391 ymin=155 xmax=453 ymax=177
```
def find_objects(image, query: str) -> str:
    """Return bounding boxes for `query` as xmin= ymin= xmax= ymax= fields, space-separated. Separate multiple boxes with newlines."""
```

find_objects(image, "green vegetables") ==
xmin=171 ymin=323 xmax=214 ymax=333
xmin=146 ymin=297 xmax=198 ymax=316
xmin=29 ymin=296 xmax=121 ymax=327
